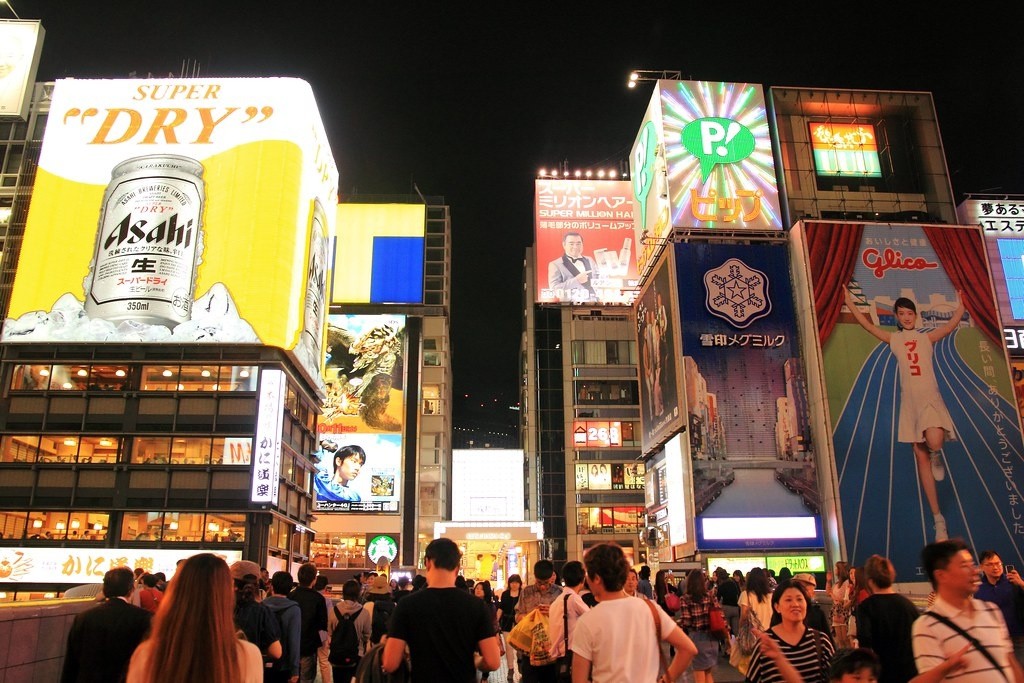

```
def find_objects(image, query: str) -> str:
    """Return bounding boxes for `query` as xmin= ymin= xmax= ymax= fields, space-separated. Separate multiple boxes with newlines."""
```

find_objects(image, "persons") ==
xmin=56 ymin=565 xmax=160 ymax=682
xmin=746 ymin=581 xmax=842 ymax=682
xmin=855 ymin=553 xmax=924 ymax=683
xmin=224 ymin=557 xmax=283 ymax=683
xmin=910 ymin=537 xmax=1024 ymax=683
xmin=324 ymin=578 xmax=372 ymax=683
xmin=285 ymin=559 xmax=330 ymax=682
xmin=824 ymin=562 xmax=851 ymax=653
xmin=845 ymin=563 xmax=872 ymax=643
xmin=976 ymin=549 xmax=1024 ymax=665
xmin=309 ymin=572 xmax=341 ymax=682
xmin=737 ymin=566 xmax=776 ymax=683
xmin=840 ymin=281 xmax=966 ymax=546
xmin=466 ymin=581 xmax=501 ymax=683
xmin=750 ymin=626 xmax=973 ymax=683
xmin=583 ymin=512 xmax=646 ymax=537
xmin=315 ymin=437 xmax=366 ymax=505
xmin=488 ymin=552 xmax=499 ymax=580
xmin=495 ymin=574 xmax=530 ymax=678
xmin=589 ymin=463 xmax=613 ymax=491
xmin=70 ymin=364 xmax=130 ymax=393
xmin=0 ymin=513 xmax=249 ymax=543
xmin=475 ymin=552 xmax=484 ymax=580
xmin=570 ymin=542 xmax=697 ymax=683
xmin=669 ymin=568 xmax=734 ymax=683
xmin=125 ymin=553 xmax=263 ymax=682
xmin=547 ymin=560 xmax=594 ymax=683
xmin=769 ymin=573 xmax=837 ymax=656
xmin=8 ymin=435 xmax=247 ymax=470
xmin=381 ymin=537 xmax=500 ymax=683
xmin=513 ymin=559 xmax=568 ymax=682
xmin=256 ymin=570 xmax=303 ymax=683
xmin=548 ymin=233 xmax=601 ymax=303
xmin=837 ymin=565 xmax=861 ymax=653
xmin=120 ymin=550 xmax=746 ymax=663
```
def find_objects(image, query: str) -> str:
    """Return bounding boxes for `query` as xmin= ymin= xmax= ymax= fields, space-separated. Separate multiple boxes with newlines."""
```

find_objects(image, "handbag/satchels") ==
xmin=737 ymin=607 xmax=765 ymax=657
xmin=529 ymin=606 xmax=559 ymax=666
xmin=506 ymin=608 xmax=536 ymax=658
xmin=665 ymin=592 xmax=680 ymax=612
xmin=709 ymin=605 xmax=729 ymax=641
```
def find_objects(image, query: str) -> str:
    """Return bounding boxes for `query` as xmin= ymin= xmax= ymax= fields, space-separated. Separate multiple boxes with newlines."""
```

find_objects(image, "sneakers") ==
xmin=933 ymin=512 xmax=948 ymax=542
xmin=507 ymin=669 xmax=515 ymax=679
xmin=929 ymin=450 xmax=945 ymax=482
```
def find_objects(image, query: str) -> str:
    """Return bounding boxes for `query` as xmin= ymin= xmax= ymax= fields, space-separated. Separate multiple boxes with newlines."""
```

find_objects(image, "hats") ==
xmin=792 ymin=573 xmax=816 ymax=587
xmin=366 ymin=576 xmax=392 ymax=595
xmin=230 ymin=560 xmax=261 ymax=583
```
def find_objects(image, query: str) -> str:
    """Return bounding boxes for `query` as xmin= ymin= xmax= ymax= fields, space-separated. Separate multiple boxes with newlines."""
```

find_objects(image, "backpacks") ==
xmin=327 ymin=606 xmax=363 ymax=665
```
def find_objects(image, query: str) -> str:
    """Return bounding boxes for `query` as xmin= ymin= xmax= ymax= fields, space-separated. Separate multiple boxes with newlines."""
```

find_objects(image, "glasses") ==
xmin=980 ymin=562 xmax=1003 ymax=566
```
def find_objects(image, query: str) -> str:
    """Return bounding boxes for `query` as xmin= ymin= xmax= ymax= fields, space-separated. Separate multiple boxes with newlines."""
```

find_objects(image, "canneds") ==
xmin=79 ymin=154 xmax=205 ymax=335
